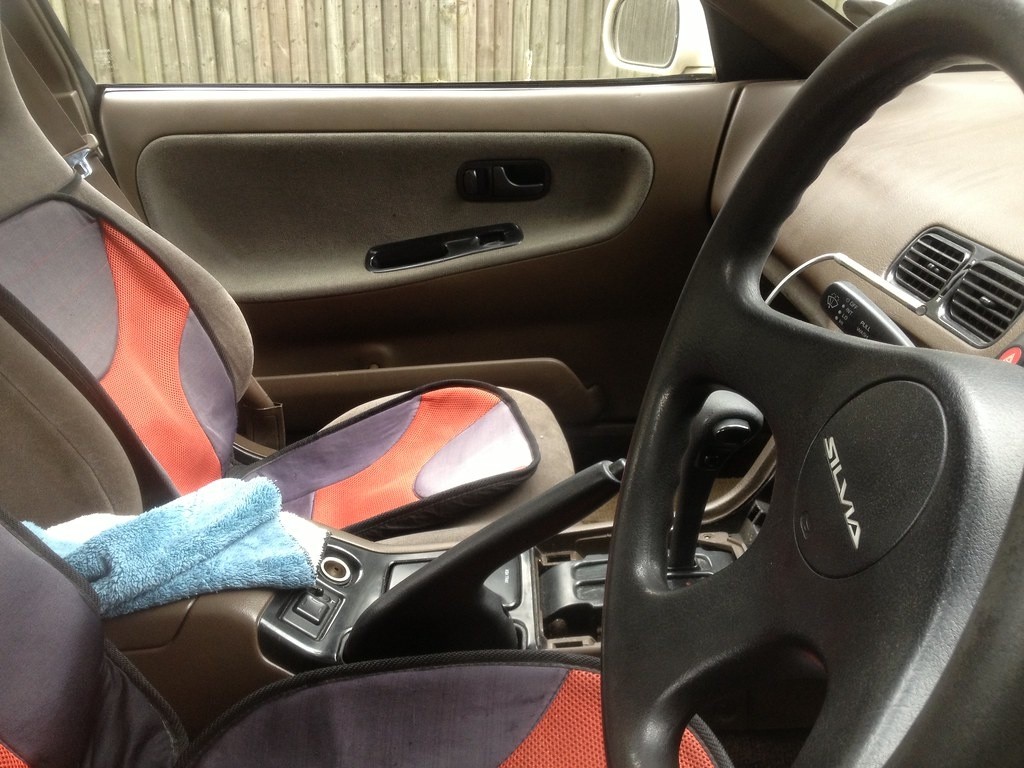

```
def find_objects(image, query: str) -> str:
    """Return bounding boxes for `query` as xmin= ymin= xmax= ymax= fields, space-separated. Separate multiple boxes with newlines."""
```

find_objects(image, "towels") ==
xmin=19 ymin=475 xmax=333 ymax=620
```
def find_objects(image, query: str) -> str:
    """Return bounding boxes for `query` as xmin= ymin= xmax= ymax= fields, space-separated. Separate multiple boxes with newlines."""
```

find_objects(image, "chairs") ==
xmin=0 ymin=511 xmax=736 ymax=768
xmin=0 ymin=38 xmax=577 ymax=548
